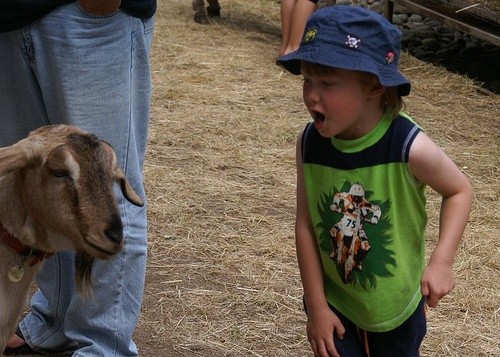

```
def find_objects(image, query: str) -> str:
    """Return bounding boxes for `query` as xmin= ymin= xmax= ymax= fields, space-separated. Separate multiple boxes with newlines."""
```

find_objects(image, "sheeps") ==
xmin=0 ymin=125 xmax=144 ymax=356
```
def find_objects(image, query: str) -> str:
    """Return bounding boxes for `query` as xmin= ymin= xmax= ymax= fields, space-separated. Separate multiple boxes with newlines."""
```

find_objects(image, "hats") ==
xmin=276 ymin=4 xmax=411 ymax=97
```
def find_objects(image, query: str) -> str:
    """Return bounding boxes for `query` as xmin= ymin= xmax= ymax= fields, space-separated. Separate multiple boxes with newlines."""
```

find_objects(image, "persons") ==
xmin=277 ymin=0 xmax=319 ymax=57
xmin=276 ymin=5 xmax=473 ymax=357
xmin=0 ymin=0 xmax=157 ymax=357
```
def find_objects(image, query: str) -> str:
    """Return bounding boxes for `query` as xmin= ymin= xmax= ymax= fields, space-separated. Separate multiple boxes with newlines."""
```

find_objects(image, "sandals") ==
xmin=4 ymin=328 xmax=34 ymax=355
